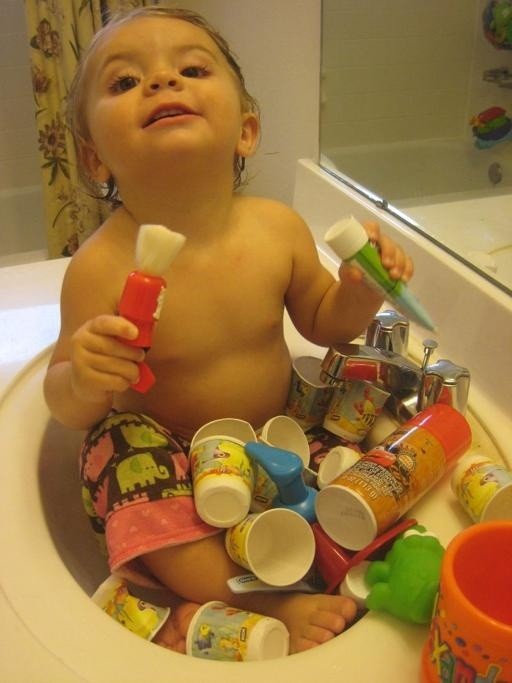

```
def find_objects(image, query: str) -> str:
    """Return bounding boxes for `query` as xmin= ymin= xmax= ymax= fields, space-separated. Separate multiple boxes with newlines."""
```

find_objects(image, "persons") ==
xmin=40 ymin=6 xmax=416 ymax=654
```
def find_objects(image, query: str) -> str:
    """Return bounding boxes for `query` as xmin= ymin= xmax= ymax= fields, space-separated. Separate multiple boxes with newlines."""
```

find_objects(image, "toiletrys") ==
xmin=324 ymin=214 xmax=440 ymax=336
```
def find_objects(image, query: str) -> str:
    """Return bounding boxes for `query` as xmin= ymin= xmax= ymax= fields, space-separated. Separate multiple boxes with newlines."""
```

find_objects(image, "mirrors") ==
xmin=315 ymin=0 xmax=511 ymax=296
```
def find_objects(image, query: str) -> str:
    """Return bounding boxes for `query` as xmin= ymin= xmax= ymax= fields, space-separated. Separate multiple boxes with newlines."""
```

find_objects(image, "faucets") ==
xmin=318 ymin=344 xmax=422 ymax=392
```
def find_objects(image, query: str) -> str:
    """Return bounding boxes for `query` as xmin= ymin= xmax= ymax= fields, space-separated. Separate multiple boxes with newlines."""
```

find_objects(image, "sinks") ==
xmin=0 ymin=303 xmax=512 ymax=683
xmin=485 ymin=243 xmax=512 ymax=281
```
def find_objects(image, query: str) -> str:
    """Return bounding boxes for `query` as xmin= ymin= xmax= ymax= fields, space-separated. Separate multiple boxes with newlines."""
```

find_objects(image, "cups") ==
xmin=323 ymin=373 xmax=391 ymax=443
xmin=225 ymin=507 xmax=318 ymax=587
xmin=449 ymin=453 xmax=512 ymax=525
xmin=185 ymin=600 xmax=291 ymax=664
xmin=187 ymin=435 xmax=257 ymax=530
xmin=253 ymin=415 xmax=310 ymax=511
xmin=313 ymin=402 xmax=472 ymax=552
xmin=90 ymin=572 xmax=171 ymax=641
xmin=192 ymin=419 xmax=257 ymax=454
xmin=315 ymin=445 xmax=364 ymax=490
xmin=284 ymin=356 xmax=334 ymax=429
